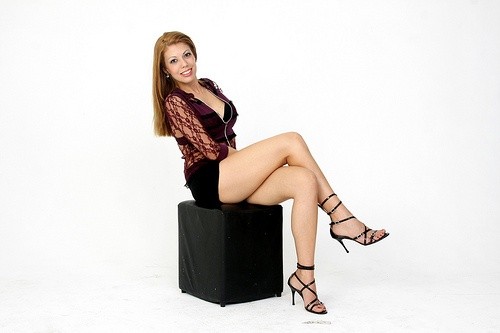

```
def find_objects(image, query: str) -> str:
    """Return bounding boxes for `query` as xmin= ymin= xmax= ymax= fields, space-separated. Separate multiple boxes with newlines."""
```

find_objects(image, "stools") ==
xmin=177 ymin=200 xmax=284 ymax=307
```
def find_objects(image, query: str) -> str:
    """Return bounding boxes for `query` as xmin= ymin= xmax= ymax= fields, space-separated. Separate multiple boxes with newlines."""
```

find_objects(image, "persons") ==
xmin=151 ymin=31 xmax=390 ymax=314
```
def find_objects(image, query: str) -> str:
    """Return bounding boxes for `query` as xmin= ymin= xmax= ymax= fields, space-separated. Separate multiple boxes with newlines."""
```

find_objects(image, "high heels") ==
xmin=288 ymin=263 xmax=328 ymax=315
xmin=318 ymin=193 xmax=390 ymax=253
